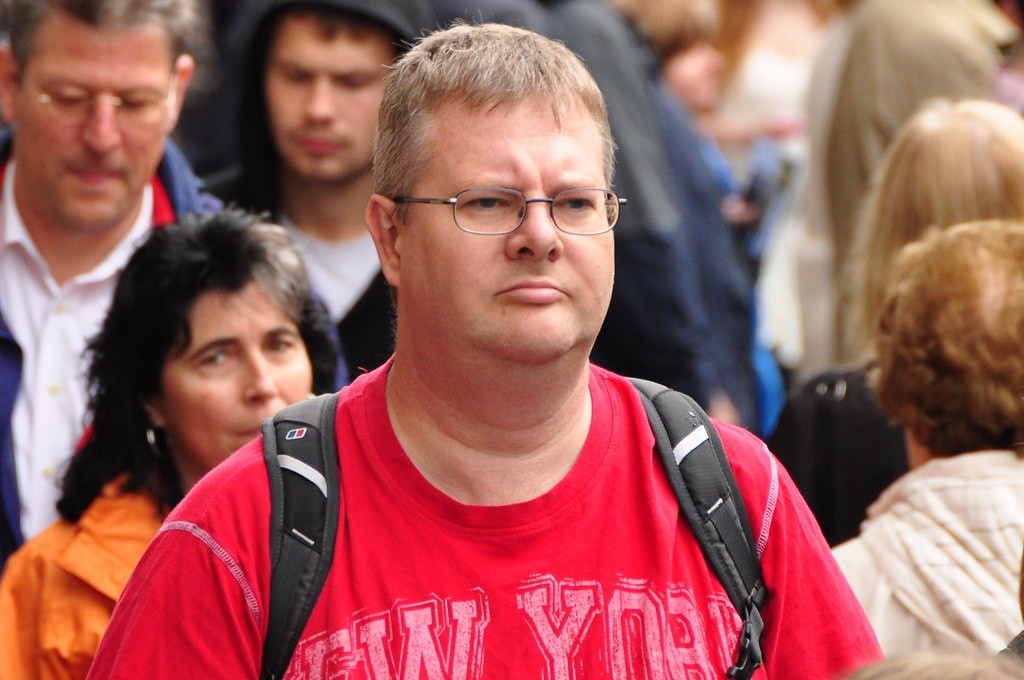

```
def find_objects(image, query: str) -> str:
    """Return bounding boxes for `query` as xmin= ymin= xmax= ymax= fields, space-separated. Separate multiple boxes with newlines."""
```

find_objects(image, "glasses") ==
xmin=392 ymin=188 xmax=627 ymax=237
xmin=16 ymin=67 xmax=177 ymax=134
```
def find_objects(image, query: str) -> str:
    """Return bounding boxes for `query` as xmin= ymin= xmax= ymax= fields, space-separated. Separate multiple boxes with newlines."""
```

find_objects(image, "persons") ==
xmin=0 ymin=0 xmax=348 ymax=583
xmin=990 ymin=549 xmax=1024 ymax=680
xmin=198 ymin=0 xmax=449 ymax=387
xmin=767 ymin=99 xmax=1024 ymax=549
xmin=804 ymin=220 xmax=1024 ymax=680
xmin=86 ymin=24 xmax=883 ymax=680
xmin=758 ymin=0 xmax=1007 ymax=398
xmin=388 ymin=0 xmax=801 ymax=443
xmin=0 ymin=205 xmax=341 ymax=680
